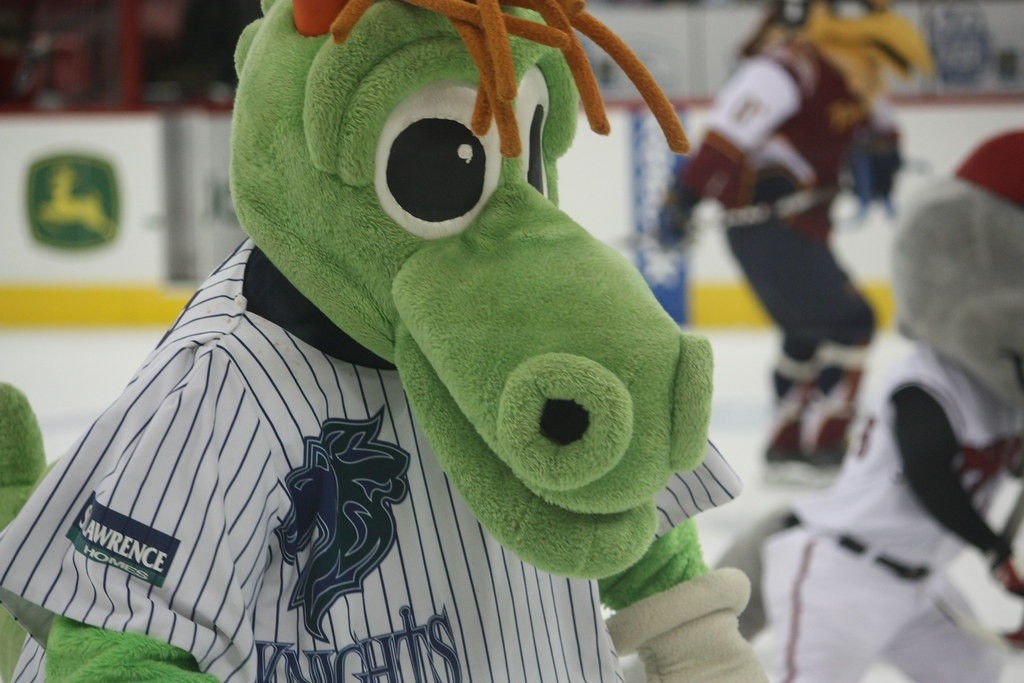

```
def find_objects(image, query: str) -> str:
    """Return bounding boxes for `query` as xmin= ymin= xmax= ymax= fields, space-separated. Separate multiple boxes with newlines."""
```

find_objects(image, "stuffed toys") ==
xmin=0 ymin=1 xmax=770 ymax=683
xmin=647 ymin=1 xmax=938 ymax=467
xmin=620 ymin=131 xmax=1024 ymax=681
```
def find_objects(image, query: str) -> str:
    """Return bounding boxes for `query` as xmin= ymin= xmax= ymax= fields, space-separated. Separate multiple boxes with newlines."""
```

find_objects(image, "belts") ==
xmin=838 ymin=535 xmax=930 ymax=580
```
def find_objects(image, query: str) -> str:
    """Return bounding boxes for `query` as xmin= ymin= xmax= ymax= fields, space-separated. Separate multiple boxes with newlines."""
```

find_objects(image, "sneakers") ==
xmin=743 ymin=448 xmax=848 ymax=506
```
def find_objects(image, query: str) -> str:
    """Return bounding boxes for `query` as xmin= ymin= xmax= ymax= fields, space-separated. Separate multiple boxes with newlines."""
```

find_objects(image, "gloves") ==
xmin=654 ymin=194 xmax=687 ymax=250
xmin=851 ymin=136 xmax=901 ymax=205
